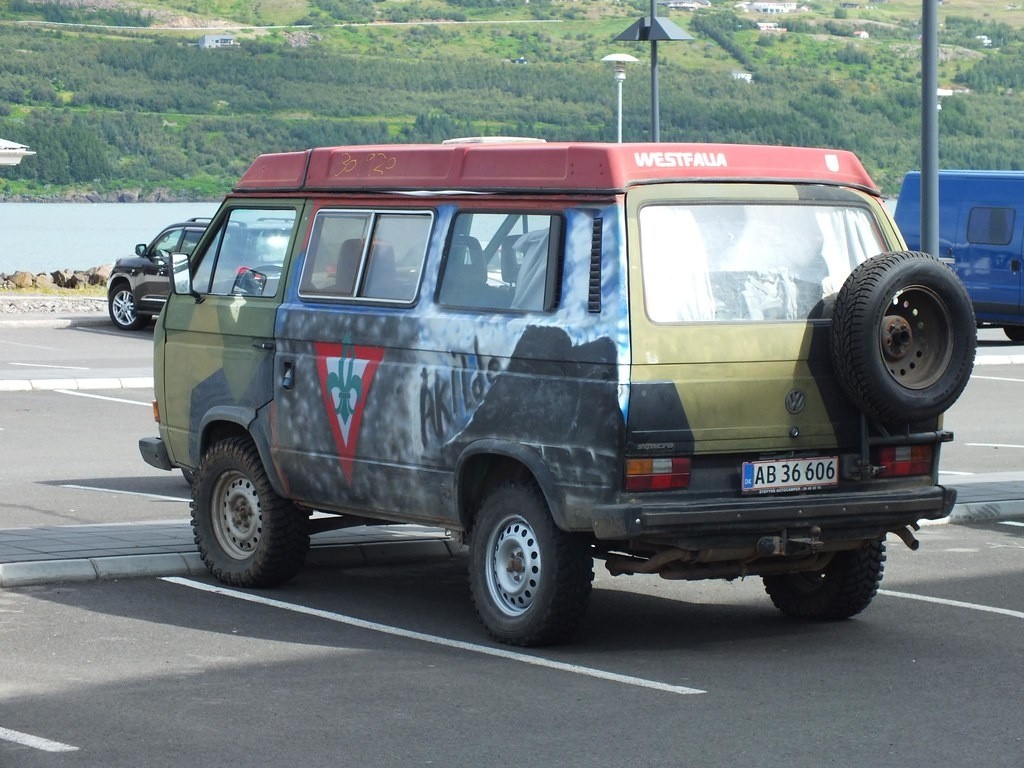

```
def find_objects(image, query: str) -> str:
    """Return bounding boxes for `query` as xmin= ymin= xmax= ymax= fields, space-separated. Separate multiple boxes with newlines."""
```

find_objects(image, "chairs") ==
xmin=456 ymin=237 xmax=506 ymax=308
xmin=499 ymin=235 xmax=527 ymax=305
xmin=321 ymin=239 xmax=409 ymax=301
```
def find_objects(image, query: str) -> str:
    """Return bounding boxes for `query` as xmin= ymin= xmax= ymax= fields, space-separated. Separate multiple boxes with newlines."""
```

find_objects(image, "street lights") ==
xmin=600 ymin=54 xmax=639 ymax=142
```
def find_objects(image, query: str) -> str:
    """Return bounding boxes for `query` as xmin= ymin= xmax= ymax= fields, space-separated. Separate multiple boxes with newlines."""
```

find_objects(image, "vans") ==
xmin=887 ymin=169 xmax=1024 ymax=345
xmin=138 ymin=137 xmax=980 ymax=643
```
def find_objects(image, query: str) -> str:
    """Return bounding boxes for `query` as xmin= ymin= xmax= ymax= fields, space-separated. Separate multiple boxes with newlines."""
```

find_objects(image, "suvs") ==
xmin=106 ymin=217 xmax=299 ymax=331
